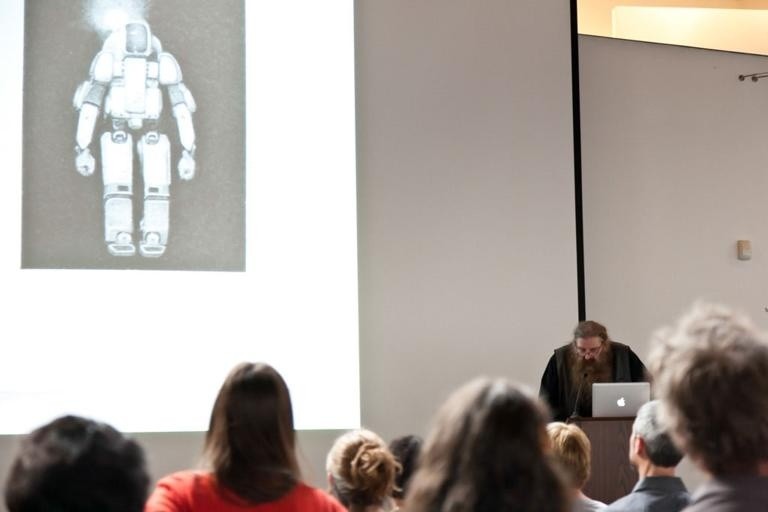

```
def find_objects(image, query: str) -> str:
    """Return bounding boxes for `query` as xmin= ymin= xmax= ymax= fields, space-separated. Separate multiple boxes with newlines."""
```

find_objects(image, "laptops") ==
xmin=592 ymin=382 xmax=650 ymax=417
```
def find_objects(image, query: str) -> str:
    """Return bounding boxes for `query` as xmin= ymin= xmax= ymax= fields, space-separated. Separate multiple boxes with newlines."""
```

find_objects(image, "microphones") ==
xmin=570 ymin=372 xmax=588 ymax=420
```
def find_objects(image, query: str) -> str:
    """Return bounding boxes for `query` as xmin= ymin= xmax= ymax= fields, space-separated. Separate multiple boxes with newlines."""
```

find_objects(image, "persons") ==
xmin=5 ymin=415 xmax=151 ymax=512
xmin=401 ymin=374 xmax=574 ymax=512
xmin=545 ymin=418 xmax=608 ymax=512
xmin=604 ymin=398 xmax=692 ymax=512
xmin=324 ymin=426 xmax=403 ymax=512
xmin=645 ymin=298 xmax=768 ymax=512
xmin=535 ymin=320 xmax=654 ymax=423
xmin=388 ymin=433 xmax=425 ymax=512
xmin=142 ymin=362 xmax=347 ymax=512
xmin=75 ymin=11 xmax=197 ymax=258
